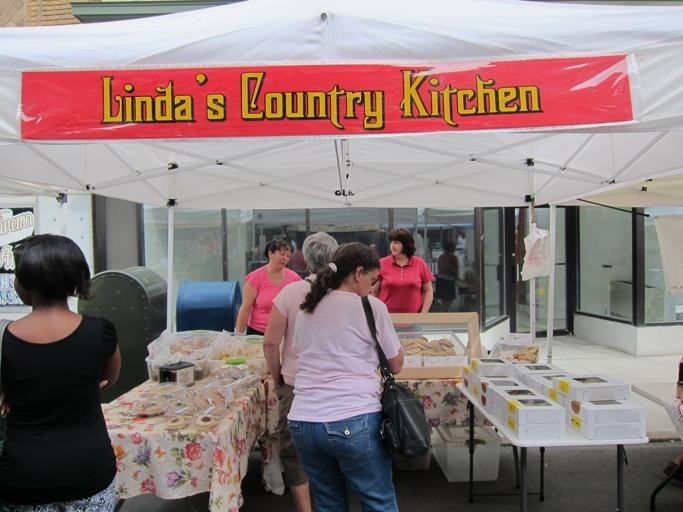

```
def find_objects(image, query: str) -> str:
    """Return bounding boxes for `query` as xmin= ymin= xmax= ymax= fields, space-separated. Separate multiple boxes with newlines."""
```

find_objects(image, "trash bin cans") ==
xmin=77 ymin=265 xmax=167 ymax=402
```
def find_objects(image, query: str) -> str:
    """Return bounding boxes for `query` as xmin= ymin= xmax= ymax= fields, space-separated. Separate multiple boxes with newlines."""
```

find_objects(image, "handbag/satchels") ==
xmin=0 ymin=391 xmax=12 ymax=458
xmin=380 ymin=381 xmax=430 ymax=472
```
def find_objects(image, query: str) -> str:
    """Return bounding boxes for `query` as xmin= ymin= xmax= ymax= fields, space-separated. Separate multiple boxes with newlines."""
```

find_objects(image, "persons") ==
xmin=231 ymin=236 xmax=304 ymax=339
xmin=0 ymin=234 xmax=123 ymax=510
xmin=431 ymin=238 xmax=460 ymax=312
xmin=369 ymin=230 xmax=390 ymax=261
xmin=285 ymin=240 xmax=405 ymax=512
xmin=360 ymin=227 xmax=436 ymax=316
xmin=261 ymin=229 xmax=342 ymax=510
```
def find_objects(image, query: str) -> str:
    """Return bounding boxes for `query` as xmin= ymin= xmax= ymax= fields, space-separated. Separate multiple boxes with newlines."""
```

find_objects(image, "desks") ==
xmin=103 ymin=338 xmax=288 ymax=512
xmin=397 ymin=378 xmax=677 ymax=512
